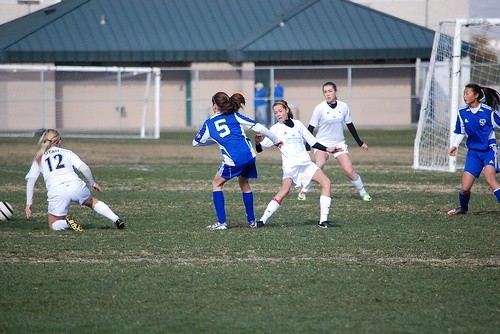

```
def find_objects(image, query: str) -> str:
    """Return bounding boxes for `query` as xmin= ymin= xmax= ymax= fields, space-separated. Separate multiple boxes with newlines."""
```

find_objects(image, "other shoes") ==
xmin=115 ymin=219 xmax=125 ymax=229
xmin=65 ymin=215 xmax=84 ymax=231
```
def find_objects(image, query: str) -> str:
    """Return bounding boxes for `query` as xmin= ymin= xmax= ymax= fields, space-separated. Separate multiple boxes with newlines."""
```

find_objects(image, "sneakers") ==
xmin=248 ymin=220 xmax=257 ymax=228
xmin=297 ymin=192 xmax=306 ymax=201
xmin=317 ymin=221 xmax=335 ymax=228
xmin=257 ymin=221 xmax=264 ymax=227
xmin=448 ymin=207 xmax=468 ymax=215
xmin=360 ymin=192 xmax=372 ymax=201
xmin=206 ymin=222 xmax=227 ymax=231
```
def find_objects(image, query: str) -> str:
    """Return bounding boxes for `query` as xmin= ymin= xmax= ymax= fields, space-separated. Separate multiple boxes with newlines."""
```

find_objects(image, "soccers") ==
xmin=0 ymin=201 xmax=14 ymax=222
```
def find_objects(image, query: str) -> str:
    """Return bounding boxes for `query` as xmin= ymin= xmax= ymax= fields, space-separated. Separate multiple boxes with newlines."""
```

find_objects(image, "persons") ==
xmin=193 ymin=82 xmax=372 ymax=229
xmin=25 ymin=129 xmax=126 ymax=231
xmin=446 ymin=84 xmax=500 ymax=216
